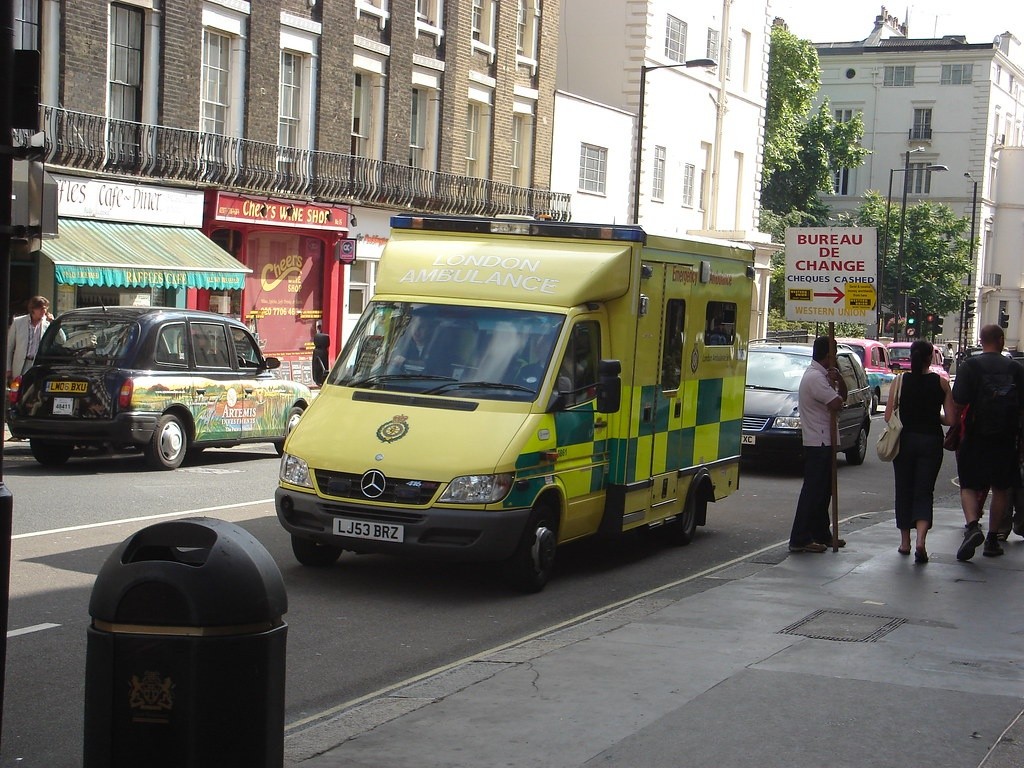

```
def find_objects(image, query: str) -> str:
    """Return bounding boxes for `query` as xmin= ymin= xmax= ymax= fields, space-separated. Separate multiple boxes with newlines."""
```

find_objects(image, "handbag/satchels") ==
xmin=876 ymin=372 xmax=905 ymax=462
xmin=944 ymin=415 xmax=963 ymax=452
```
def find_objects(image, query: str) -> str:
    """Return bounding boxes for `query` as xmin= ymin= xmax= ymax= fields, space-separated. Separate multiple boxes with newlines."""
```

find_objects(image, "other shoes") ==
xmin=957 ymin=528 xmax=984 ymax=562
xmin=817 ymin=537 xmax=846 ymax=547
xmin=983 ymin=538 xmax=1004 ymax=557
xmin=789 ymin=540 xmax=827 ymax=552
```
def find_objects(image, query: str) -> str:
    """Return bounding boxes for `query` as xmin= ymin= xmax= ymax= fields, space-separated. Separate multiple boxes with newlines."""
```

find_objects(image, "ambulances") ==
xmin=272 ymin=208 xmax=758 ymax=596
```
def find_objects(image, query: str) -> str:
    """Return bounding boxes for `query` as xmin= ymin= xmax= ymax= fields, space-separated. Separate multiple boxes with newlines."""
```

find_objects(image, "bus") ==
xmin=887 ymin=341 xmax=951 ymax=388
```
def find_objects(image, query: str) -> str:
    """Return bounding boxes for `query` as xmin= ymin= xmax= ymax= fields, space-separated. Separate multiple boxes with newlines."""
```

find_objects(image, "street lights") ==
xmin=631 ymin=58 xmax=722 ymax=226
xmin=892 ymin=145 xmax=927 ymax=342
xmin=876 ymin=164 xmax=950 ymax=337
xmin=963 ymin=172 xmax=978 ymax=356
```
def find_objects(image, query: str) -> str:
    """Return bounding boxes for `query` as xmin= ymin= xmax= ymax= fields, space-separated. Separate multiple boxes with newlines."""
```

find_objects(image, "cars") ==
xmin=739 ymin=341 xmax=875 ymax=465
xmin=834 ymin=337 xmax=902 ymax=415
xmin=6 ymin=306 xmax=314 ymax=471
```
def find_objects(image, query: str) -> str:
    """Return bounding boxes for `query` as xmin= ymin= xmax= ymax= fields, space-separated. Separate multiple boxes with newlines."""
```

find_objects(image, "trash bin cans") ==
xmin=83 ymin=517 xmax=289 ymax=768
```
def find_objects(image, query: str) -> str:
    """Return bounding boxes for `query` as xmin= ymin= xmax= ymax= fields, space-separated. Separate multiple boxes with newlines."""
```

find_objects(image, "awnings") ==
xmin=40 ymin=215 xmax=254 ymax=290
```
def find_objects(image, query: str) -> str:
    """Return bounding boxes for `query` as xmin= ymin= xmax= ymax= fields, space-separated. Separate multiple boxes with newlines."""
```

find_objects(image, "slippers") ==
xmin=899 ymin=544 xmax=910 ymax=555
xmin=914 ymin=547 xmax=929 ymax=563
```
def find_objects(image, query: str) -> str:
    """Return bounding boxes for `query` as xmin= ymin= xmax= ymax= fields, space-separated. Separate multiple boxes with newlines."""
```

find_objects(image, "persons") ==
xmin=789 ymin=336 xmax=848 ymax=552
xmin=952 ymin=325 xmax=1024 ymax=560
xmin=5 ymin=296 xmax=68 ymax=441
xmin=883 ymin=340 xmax=957 ymax=563
xmin=942 ymin=343 xmax=954 ymax=373
xmin=384 ymin=312 xmax=581 ymax=407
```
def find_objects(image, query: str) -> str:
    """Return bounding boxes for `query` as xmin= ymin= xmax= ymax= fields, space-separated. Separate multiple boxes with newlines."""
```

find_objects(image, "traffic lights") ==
xmin=933 ymin=314 xmax=943 ymax=337
xmin=963 ymin=299 xmax=976 ymax=330
xmin=1000 ymin=307 xmax=1010 ymax=329
xmin=926 ymin=312 xmax=935 ymax=332
xmin=905 ymin=296 xmax=922 ymax=336
